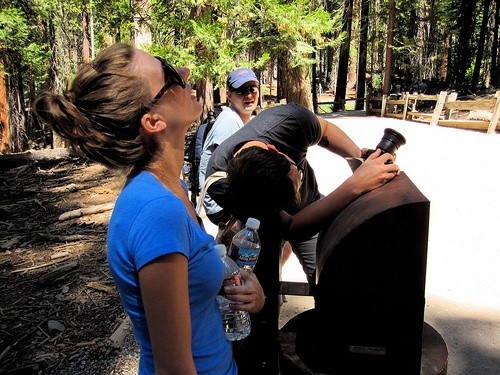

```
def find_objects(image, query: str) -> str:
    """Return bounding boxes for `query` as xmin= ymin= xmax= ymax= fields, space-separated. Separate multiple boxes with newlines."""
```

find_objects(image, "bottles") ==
xmin=216 ymin=217 xmax=262 ymax=304
xmin=213 ymin=244 xmax=251 ymax=342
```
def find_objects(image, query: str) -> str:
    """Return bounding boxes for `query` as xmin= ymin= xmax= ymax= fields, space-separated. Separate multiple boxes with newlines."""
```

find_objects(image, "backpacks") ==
xmin=184 ymin=110 xmax=217 ymax=196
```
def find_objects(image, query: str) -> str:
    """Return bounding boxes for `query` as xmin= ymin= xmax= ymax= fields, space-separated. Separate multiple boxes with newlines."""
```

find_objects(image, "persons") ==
xmin=34 ymin=42 xmax=265 ymax=375
xmin=199 ymin=68 xmax=292 ymax=303
xmin=205 ymin=101 xmax=401 ymax=375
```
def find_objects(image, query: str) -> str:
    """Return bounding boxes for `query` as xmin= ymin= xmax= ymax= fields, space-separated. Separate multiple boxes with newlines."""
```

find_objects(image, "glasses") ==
xmin=232 ymin=85 xmax=258 ymax=95
xmin=138 ymin=56 xmax=186 ymax=114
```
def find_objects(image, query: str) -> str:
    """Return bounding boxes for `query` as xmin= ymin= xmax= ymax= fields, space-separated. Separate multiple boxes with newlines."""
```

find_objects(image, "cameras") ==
xmin=364 ymin=128 xmax=405 ymax=164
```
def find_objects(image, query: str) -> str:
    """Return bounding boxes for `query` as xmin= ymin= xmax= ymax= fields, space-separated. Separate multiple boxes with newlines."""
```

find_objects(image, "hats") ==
xmin=227 ymin=68 xmax=259 ymax=88
